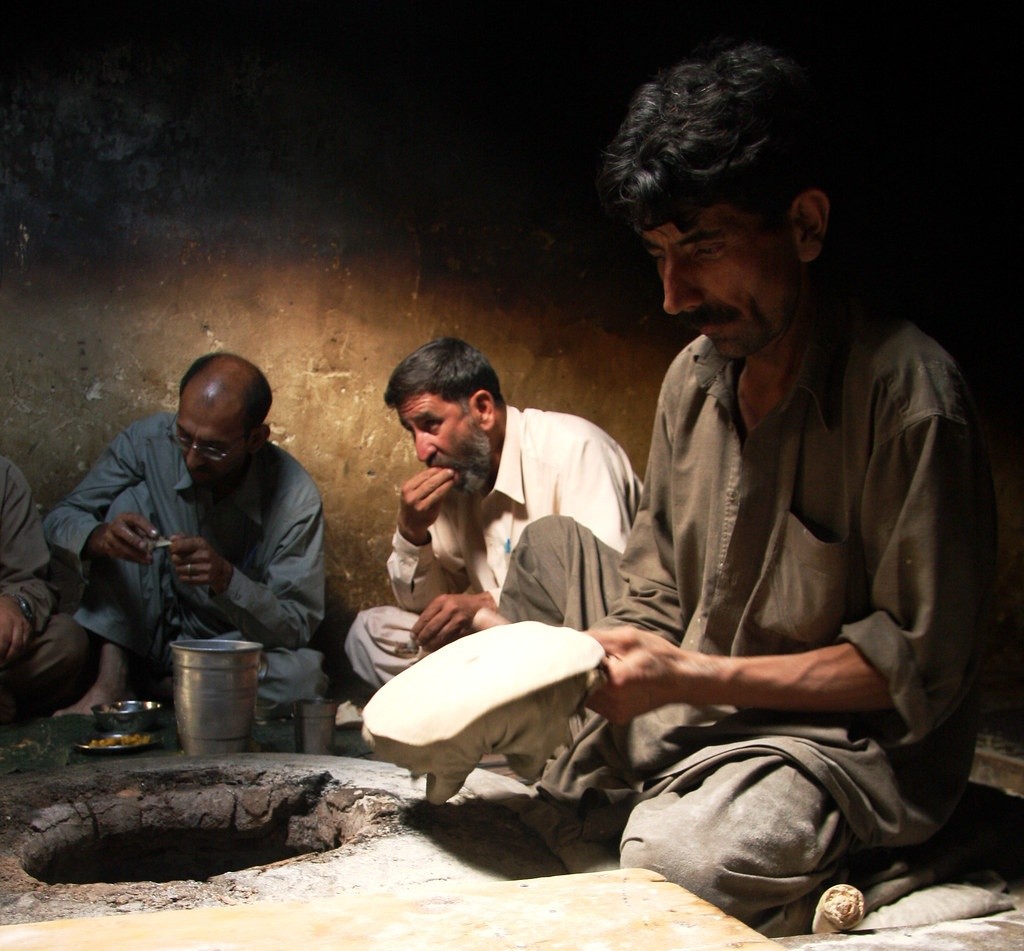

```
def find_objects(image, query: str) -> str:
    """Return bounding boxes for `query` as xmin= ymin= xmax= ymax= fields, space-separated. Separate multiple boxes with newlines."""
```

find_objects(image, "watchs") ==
xmin=7 ymin=593 xmax=35 ymax=624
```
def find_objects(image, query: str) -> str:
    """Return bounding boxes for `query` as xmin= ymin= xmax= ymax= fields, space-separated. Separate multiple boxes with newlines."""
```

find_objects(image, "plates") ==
xmin=73 ymin=731 xmax=162 ymax=751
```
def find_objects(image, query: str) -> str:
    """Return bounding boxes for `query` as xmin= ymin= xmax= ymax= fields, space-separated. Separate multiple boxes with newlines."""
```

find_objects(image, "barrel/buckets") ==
xmin=170 ymin=641 xmax=263 ymax=741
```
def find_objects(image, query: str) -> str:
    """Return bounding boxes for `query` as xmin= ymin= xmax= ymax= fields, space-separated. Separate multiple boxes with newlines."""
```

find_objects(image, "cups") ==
xmin=295 ymin=698 xmax=336 ymax=755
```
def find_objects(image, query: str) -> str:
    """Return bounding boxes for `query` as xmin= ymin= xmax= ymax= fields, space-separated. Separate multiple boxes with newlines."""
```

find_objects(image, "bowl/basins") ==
xmin=92 ymin=699 xmax=161 ymax=730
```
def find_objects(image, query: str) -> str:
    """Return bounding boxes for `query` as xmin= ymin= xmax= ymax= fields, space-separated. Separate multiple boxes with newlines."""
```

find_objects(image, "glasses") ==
xmin=165 ymin=408 xmax=249 ymax=463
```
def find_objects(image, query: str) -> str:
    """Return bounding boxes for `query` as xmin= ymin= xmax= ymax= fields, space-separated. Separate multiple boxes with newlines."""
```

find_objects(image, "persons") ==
xmin=43 ymin=353 xmax=331 ymax=723
xmin=491 ymin=34 xmax=1021 ymax=939
xmin=345 ymin=336 xmax=650 ymax=695
xmin=0 ymin=456 xmax=86 ymax=718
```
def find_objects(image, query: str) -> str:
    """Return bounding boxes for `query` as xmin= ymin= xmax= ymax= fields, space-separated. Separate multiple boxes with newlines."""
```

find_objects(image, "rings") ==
xmin=185 ymin=563 xmax=193 ymax=576
